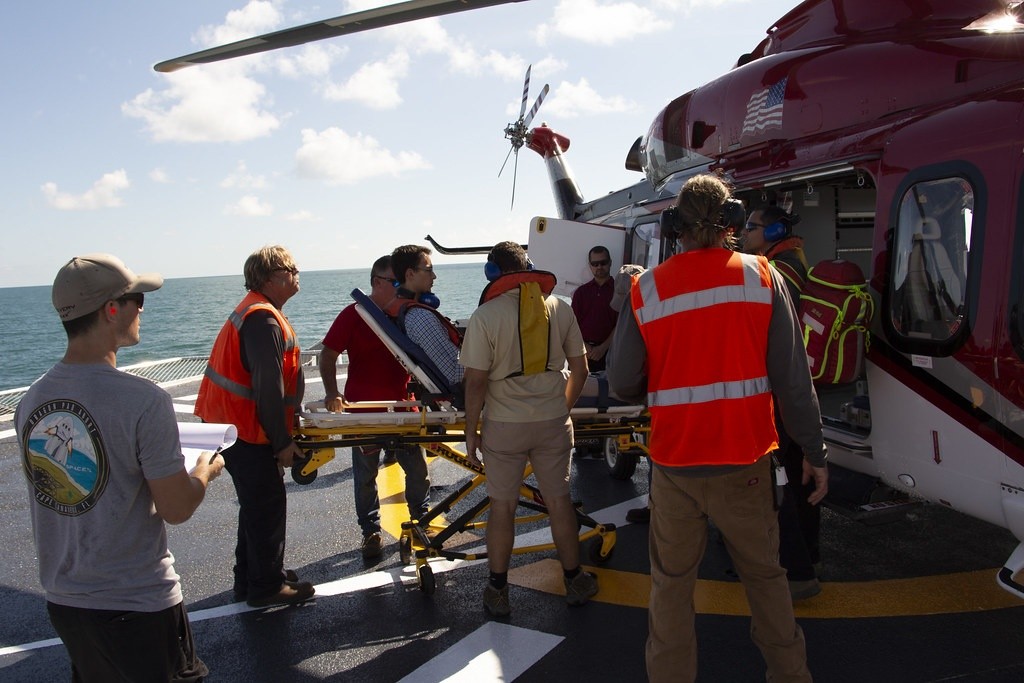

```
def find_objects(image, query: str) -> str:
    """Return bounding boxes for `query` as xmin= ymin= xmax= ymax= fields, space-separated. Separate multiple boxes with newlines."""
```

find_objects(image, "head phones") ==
xmin=763 ymin=214 xmax=802 ymax=243
xmin=659 ymin=199 xmax=747 ymax=240
xmin=397 ymin=287 xmax=441 ymax=310
xmin=484 ymin=250 xmax=534 ymax=282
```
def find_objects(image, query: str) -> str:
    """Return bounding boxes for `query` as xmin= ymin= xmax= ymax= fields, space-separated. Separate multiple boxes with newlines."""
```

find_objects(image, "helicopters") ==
xmin=152 ymin=1 xmax=1023 ymax=598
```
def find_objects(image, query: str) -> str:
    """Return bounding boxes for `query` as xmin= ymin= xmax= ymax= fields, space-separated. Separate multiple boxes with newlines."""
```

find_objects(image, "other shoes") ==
xmin=809 ymin=558 xmax=823 ymax=573
xmin=412 ymin=528 xmax=438 ymax=547
xmin=362 ymin=534 xmax=383 ymax=556
xmin=787 ymin=574 xmax=820 ymax=601
xmin=247 ymin=581 xmax=315 ymax=607
xmin=239 ymin=570 xmax=299 ymax=600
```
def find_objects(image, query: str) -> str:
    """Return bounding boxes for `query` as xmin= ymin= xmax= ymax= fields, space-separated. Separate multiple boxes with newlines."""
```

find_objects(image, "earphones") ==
xmin=110 ymin=307 xmax=116 ymax=314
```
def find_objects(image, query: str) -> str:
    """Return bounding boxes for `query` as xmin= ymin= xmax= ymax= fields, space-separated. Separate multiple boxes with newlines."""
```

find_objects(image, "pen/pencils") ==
xmin=209 ymin=446 xmax=223 ymax=464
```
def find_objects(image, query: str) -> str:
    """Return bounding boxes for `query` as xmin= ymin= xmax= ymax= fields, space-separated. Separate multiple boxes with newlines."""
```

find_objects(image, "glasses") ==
xmin=372 ymin=275 xmax=400 ymax=288
xmin=274 ymin=264 xmax=294 ymax=275
xmin=412 ymin=264 xmax=433 ymax=275
xmin=589 ymin=257 xmax=611 ymax=267
xmin=745 ymin=222 xmax=767 ymax=232
xmin=114 ymin=293 xmax=145 ymax=310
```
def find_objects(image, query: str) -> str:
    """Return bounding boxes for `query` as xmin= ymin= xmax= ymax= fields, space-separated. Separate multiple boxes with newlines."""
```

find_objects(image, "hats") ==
xmin=610 ymin=264 xmax=648 ymax=313
xmin=52 ymin=257 xmax=164 ymax=321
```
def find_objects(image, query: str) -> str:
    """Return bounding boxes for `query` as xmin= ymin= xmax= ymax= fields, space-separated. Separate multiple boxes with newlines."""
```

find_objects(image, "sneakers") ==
xmin=484 ymin=583 xmax=509 ymax=614
xmin=564 ymin=569 xmax=596 ymax=605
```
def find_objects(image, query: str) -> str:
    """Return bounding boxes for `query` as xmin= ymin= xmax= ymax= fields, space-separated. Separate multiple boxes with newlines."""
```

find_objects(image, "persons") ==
xmin=382 ymin=246 xmax=618 ymax=397
xmin=317 ymin=256 xmax=437 ymax=556
xmin=14 ymin=257 xmax=225 ymax=683
xmin=457 ymin=242 xmax=600 ymax=616
xmin=605 ymin=174 xmax=829 ymax=683
xmin=571 ymin=245 xmax=619 ymax=459
xmin=738 ymin=204 xmax=823 ymax=601
xmin=194 ymin=247 xmax=314 ymax=606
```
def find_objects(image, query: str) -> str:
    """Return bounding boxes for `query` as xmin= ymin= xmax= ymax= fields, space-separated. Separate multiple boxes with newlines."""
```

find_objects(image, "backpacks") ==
xmin=767 ymin=254 xmax=872 ymax=385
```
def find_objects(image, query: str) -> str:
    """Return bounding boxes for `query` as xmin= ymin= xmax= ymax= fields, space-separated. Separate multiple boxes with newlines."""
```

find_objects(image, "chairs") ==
xmin=904 ymin=215 xmax=963 ymax=338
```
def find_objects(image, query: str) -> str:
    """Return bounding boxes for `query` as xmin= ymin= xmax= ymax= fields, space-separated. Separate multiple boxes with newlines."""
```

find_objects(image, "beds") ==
xmin=295 ymin=288 xmax=650 ymax=428
